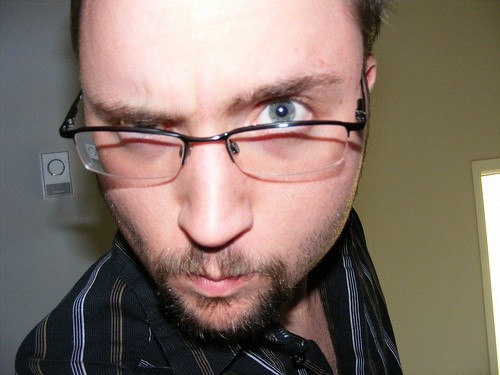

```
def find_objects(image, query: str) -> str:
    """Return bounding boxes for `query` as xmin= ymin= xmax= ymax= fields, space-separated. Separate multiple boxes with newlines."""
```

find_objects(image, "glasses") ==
xmin=56 ymin=69 xmax=368 ymax=180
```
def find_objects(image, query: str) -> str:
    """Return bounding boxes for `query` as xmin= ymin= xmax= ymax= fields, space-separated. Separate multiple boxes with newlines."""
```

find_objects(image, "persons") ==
xmin=13 ymin=0 xmax=406 ymax=375
